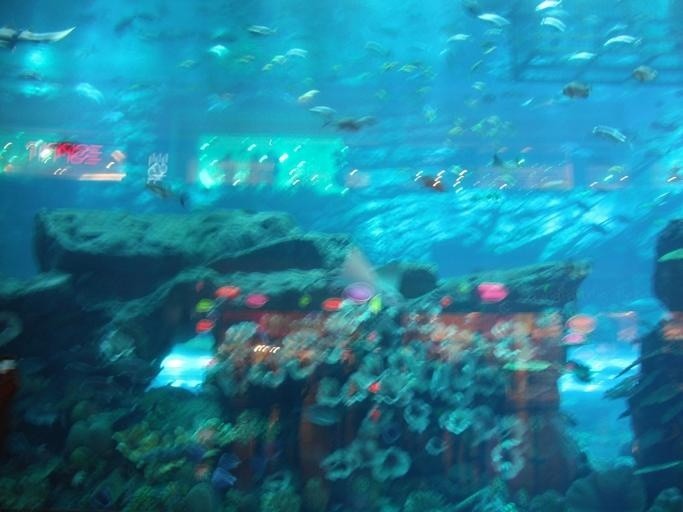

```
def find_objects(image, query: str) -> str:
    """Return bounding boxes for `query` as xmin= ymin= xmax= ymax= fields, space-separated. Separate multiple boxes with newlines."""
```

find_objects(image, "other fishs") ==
xmin=0 ymin=0 xmax=683 ymax=511
